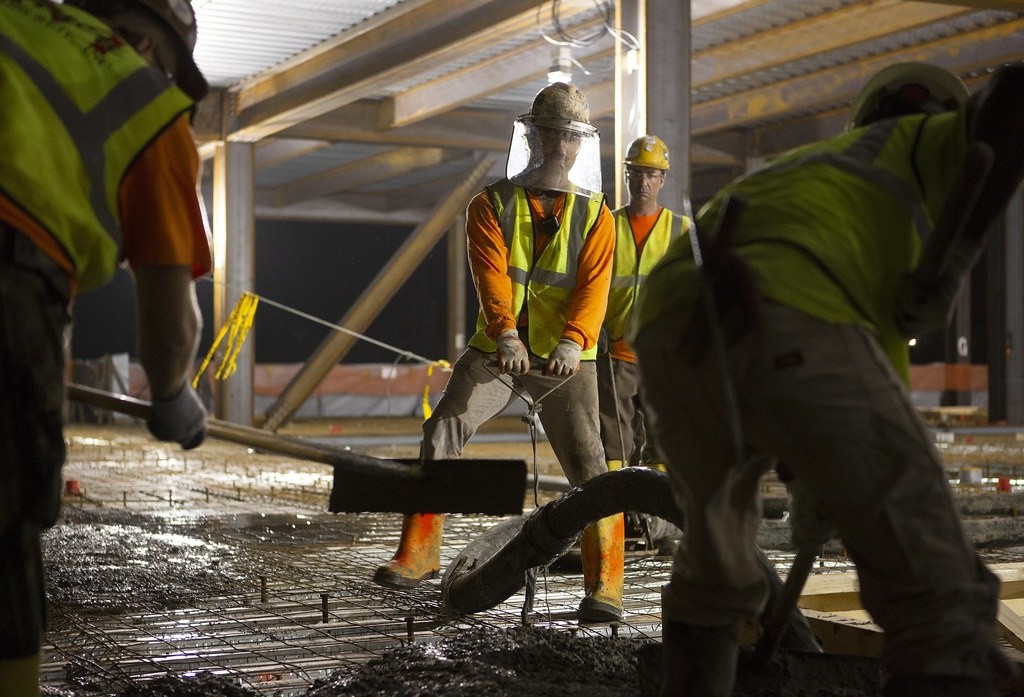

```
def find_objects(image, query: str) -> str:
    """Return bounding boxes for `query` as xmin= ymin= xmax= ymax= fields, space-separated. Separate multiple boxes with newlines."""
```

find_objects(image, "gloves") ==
xmin=541 ymin=339 xmax=581 ymax=378
xmin=598 ymin=327 xmax=613 ymax=354
xmin=496 ymin=328 xmax=531 ymax=376
xmin=145 ymin=378 xmax=206 ymax=450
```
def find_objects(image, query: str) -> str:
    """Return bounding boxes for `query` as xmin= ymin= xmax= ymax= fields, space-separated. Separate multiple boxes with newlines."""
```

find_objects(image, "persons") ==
xmin=597 ymin=134 xmax=693 ymax=551
xmin=373 ymin=82 xmax=625 ymax=623
xmin=0 ymin=0 xmax=215 ymax=697
xmin=630 ymin=60 xmax=1024 ymax=697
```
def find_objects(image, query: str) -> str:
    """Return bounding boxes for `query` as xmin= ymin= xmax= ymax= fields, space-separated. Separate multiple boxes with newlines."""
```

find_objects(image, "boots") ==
xmin=1 ymin=650 xmax=41 ymax=696
xmin=374 ymin=513 xmax=441 ymax=588
xmin=579 ymin=510 xmax=625 ymax=620
xmin=657 ymin=619 xmax=737 ymax=696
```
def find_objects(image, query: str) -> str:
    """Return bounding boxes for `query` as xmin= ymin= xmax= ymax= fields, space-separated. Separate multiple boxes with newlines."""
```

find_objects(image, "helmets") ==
xmin=63 ymin=0 xmax=211 ymax=103
xmin=623 ymin=133 xmax=674 ymax=173
xmin=845 ymin=61 xmax=972 ymax=131
xmin=520 ymin=82 xmax=608 ymax=138
